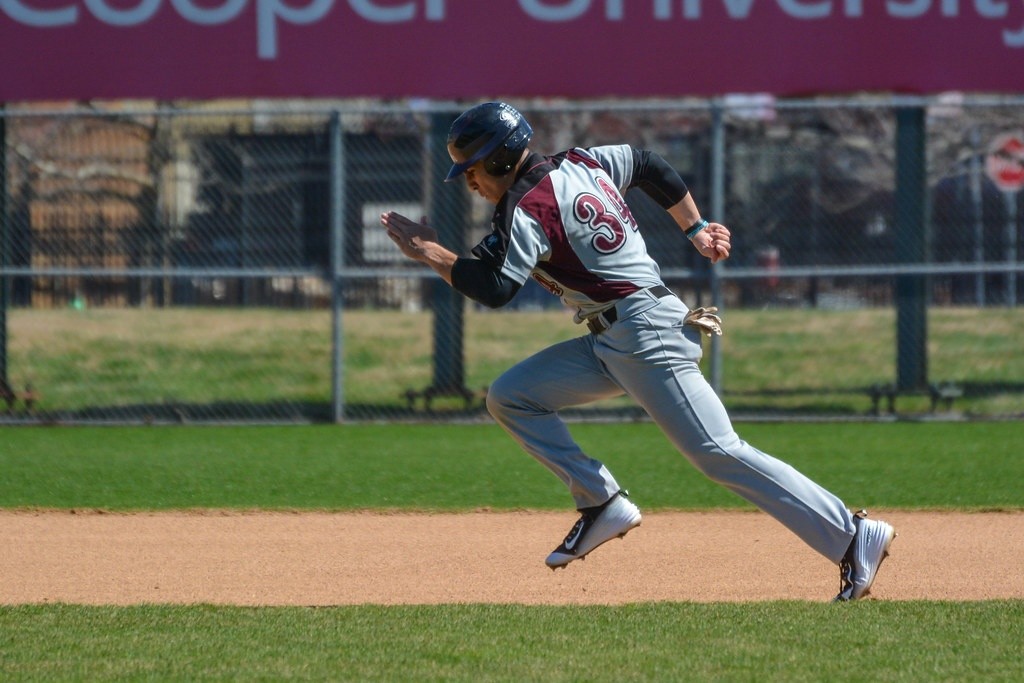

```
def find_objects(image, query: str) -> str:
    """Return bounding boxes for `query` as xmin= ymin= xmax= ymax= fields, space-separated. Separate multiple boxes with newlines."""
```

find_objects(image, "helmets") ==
xmin=443 ymin=102 xmax=534 ymax=183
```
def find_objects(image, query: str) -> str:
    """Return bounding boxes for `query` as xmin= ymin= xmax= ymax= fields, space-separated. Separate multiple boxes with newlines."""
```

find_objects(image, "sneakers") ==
xmin=545 ymin=489 xmax=641 ymax=569
xmin=835 ymin=507 xmax=897 ymax=604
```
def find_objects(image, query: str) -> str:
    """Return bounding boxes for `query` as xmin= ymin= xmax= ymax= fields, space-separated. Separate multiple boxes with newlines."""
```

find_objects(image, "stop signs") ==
xmin=986 ymin=132 xmax=1024 ymax=193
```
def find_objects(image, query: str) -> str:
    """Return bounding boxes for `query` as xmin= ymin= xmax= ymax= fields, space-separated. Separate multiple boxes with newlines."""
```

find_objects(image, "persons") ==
xmin=379 ymin=102 xmax=897 ymax=600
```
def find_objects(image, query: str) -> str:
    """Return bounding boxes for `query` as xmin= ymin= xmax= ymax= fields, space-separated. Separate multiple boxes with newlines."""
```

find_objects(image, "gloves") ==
xmin=683 ymin=306 xmax=723 ymax=337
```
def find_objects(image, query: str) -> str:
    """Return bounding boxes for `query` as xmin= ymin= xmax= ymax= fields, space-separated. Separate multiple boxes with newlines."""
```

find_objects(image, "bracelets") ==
xmin=685 ymin=218 xmax=708 ymax=239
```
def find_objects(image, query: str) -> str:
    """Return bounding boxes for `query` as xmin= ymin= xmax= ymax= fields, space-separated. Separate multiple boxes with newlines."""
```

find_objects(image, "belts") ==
xmin=587 ymin=285 xmax=671 ymax=336
